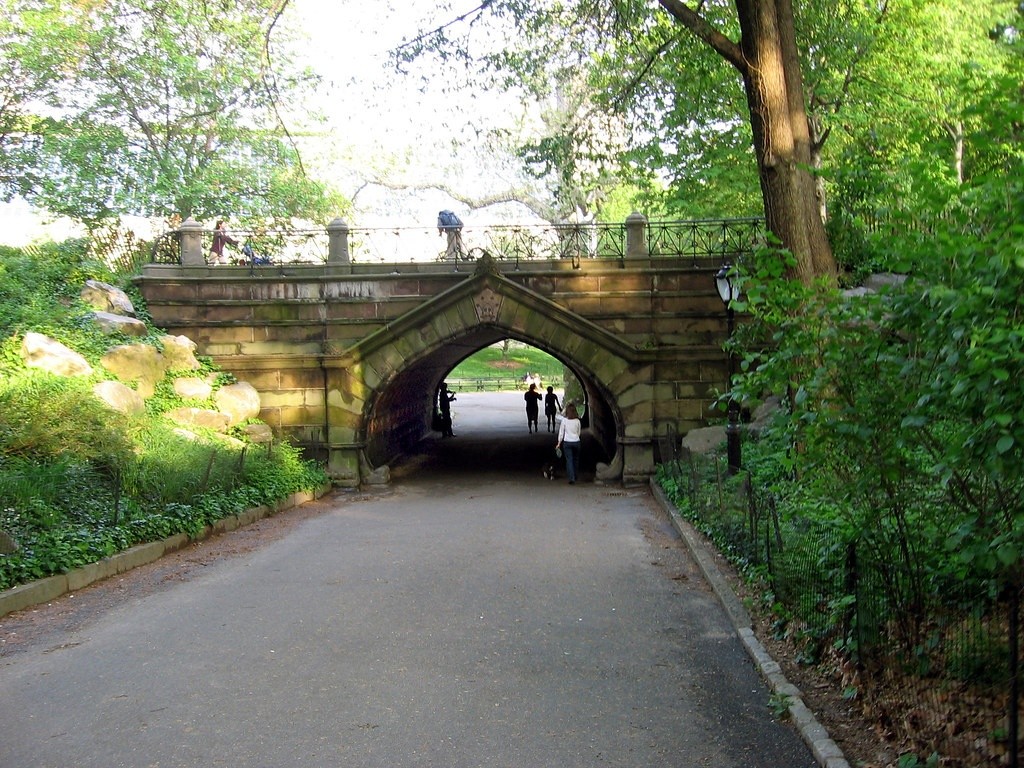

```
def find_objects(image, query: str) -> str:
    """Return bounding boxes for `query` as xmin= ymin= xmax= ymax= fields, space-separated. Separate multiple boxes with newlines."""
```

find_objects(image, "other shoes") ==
xmin=450 ymin=433 xmax=457 ymax=437
xmin=575 ymin=475 xmax=581 ymax=481
xmin=569 ymin=480 xmax=573 ymax=484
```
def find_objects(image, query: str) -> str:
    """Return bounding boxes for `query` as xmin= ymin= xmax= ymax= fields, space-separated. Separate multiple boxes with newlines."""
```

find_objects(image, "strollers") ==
xmin=232 ymin=238 xmax=274 ymax=266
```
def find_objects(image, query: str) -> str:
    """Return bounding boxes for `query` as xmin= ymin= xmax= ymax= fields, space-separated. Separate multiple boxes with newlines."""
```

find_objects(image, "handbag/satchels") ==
xmin=555 ymin=448 xmax=562 ymax=457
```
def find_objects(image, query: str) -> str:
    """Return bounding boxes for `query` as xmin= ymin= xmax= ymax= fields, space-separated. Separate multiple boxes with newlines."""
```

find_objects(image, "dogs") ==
xmin=544 ymin=465 xmax=560 ymax=480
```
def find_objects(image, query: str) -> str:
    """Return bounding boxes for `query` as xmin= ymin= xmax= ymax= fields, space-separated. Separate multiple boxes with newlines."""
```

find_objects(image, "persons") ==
xmin=240 ymin=260 xmax=245 ymax=265
xmin=552 ymin=377 xmax=560 ymax=388
xmin=438 ymin=210 xmax=466 ymax=261
xmin=440 ymin=383 xmax=456 ymax=437
xmin=210 ymin=221 xmax=240 ymax=265
xmin=524 ymin=384 xmax=542 ymax=434
xmin=556 ymin=404 xmax=581 ymax=484
xmin=526 ymin=376 xmax=541 ymax=391
xmin=516 ymin=380 xmax=524 ymax=390
xmin=458 ymin=380 xmax=503 ymax=392
xmin=545 ymin=386 xmax=562 ymax=433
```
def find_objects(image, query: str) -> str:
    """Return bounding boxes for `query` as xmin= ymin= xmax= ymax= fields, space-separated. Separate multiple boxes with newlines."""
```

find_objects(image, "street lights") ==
xmin=713 ymin=260 xmax=746 ymax=480
